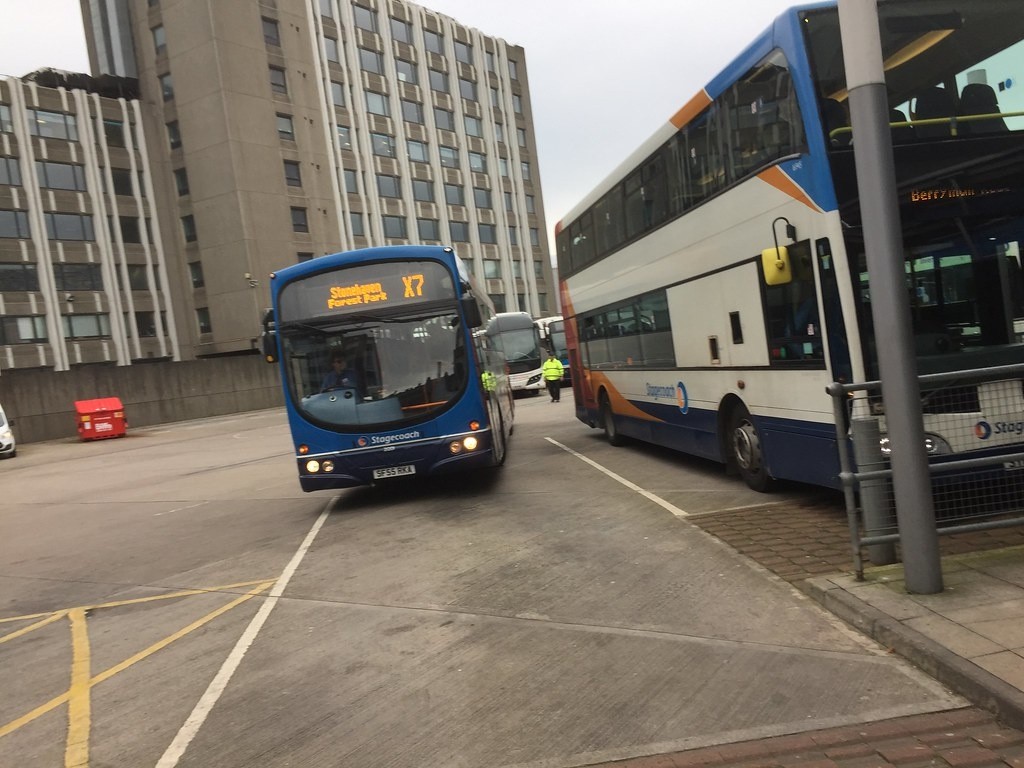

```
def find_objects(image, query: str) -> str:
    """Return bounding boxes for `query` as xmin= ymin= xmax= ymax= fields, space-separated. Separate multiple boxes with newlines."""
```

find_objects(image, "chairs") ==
xmin=607 ymin=316 xmax=654 ymax=337
xmin=734 ymin=85 xmax=1008 ymax=178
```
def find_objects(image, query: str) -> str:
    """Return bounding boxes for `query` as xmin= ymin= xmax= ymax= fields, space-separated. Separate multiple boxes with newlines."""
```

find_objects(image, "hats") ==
xmin=549 ymin=352 xmax=555 ymax=355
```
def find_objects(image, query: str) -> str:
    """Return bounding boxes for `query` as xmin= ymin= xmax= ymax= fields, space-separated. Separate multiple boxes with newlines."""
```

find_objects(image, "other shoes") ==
xmin=556 ymin=400 xmax=560 ymax=402
xmin=551 ymin=399 xmax=554 ymax=402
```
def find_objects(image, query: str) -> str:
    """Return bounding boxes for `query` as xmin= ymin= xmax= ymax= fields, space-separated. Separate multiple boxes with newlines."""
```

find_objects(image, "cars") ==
xmin=1 ymin=404 xmax=17 ymax=461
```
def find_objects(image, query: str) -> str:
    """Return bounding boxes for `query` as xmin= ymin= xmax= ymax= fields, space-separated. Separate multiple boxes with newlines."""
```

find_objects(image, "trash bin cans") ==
xmin=72 ymin=396 xmax=127 ymax=440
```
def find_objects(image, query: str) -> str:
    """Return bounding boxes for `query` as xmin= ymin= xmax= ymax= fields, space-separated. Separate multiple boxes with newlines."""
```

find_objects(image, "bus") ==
xmin=532 ymin=314 xmax=571 ymax=388
xmin=486 ymin=312 xmax=547 ymax=399
xmin=554 ymin=0 xmax=1024 ymax=501
xmin=259 ymin=244 xmax=515 ymax=494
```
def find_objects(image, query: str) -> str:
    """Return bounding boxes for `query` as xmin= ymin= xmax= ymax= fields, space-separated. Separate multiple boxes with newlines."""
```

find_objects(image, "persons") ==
xmin=320 ymin=352 xmax=356 ymax=393
xmin=542 ymin=351 xmax=564 ymax=403
xmin=481 ymin=366 xmax=498 ymax=411
